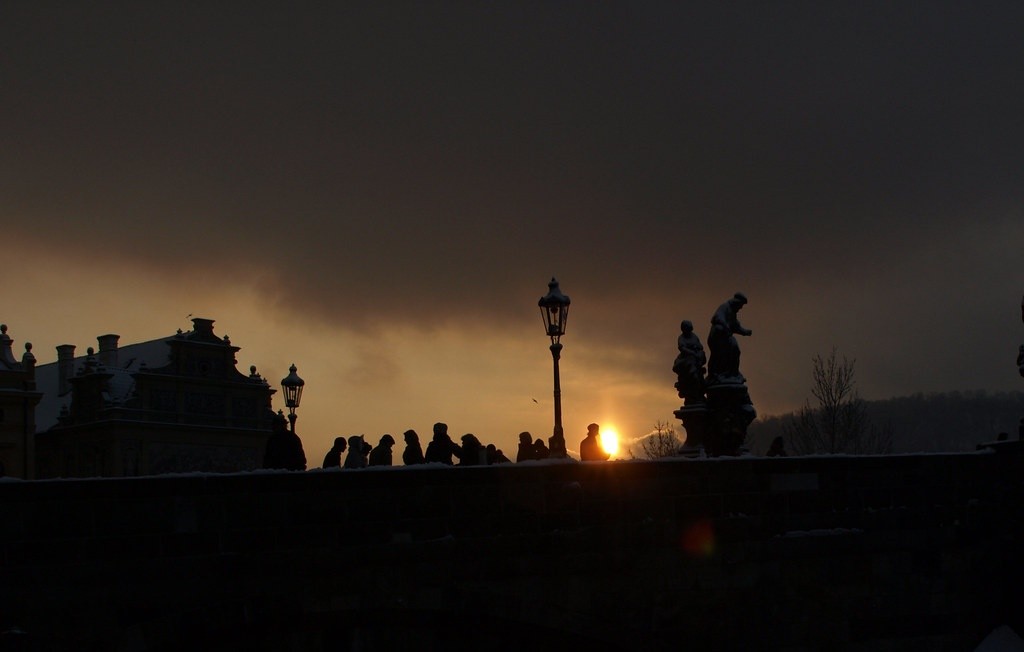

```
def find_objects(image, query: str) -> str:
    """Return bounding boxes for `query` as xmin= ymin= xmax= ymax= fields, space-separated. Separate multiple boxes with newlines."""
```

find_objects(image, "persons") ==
xmin=672 ymin=319 xmax=707 ymax=398
xmin=766 ymin=436 xmax=789 ymax=457
xmin=708 ymin=293 xmax=752 ymax=381
xmin=580 ymin=423 xmax=611 ymax=462
xmin=263 ymin=416 xmax=306 ymax=471
xmin=322 ymin=423 xmax=552 ymax=470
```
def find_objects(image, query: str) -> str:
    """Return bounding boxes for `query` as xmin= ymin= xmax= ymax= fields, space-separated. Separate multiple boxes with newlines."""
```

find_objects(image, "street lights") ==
xmin=277 ymin=409 xmax=289 ymax=429
xmin=222 ymin=335 xmax=241 ymax=365
xmin=537 ymin=278 xmax=571 ymax=459
xmin=262 ymin=377 xmax=278 ymax=411
xmin=281 ymin=363 xmax=304 ymax=433
xmin=248 ymin=366 xmax=261 ymax=379
